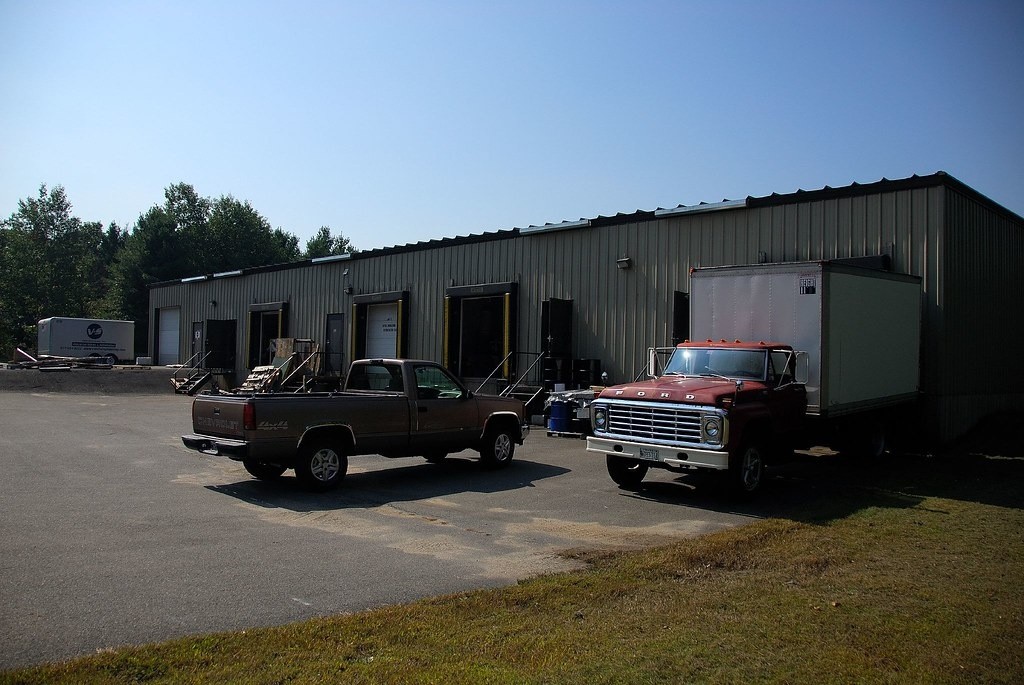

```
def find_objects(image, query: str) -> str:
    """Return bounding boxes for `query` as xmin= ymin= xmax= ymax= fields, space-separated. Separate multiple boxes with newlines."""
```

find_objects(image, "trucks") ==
xmin=589 ymin=264 xmax=923 ymax=499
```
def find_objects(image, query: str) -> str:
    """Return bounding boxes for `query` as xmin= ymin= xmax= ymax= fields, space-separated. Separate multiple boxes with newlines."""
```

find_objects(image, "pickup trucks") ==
xmin=181 ymin=359 xmax=529 ymax=489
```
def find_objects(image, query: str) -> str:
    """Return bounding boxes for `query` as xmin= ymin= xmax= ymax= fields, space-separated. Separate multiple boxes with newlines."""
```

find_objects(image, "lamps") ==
xmin=616 ymin=258 xmax=632 ymax=269
